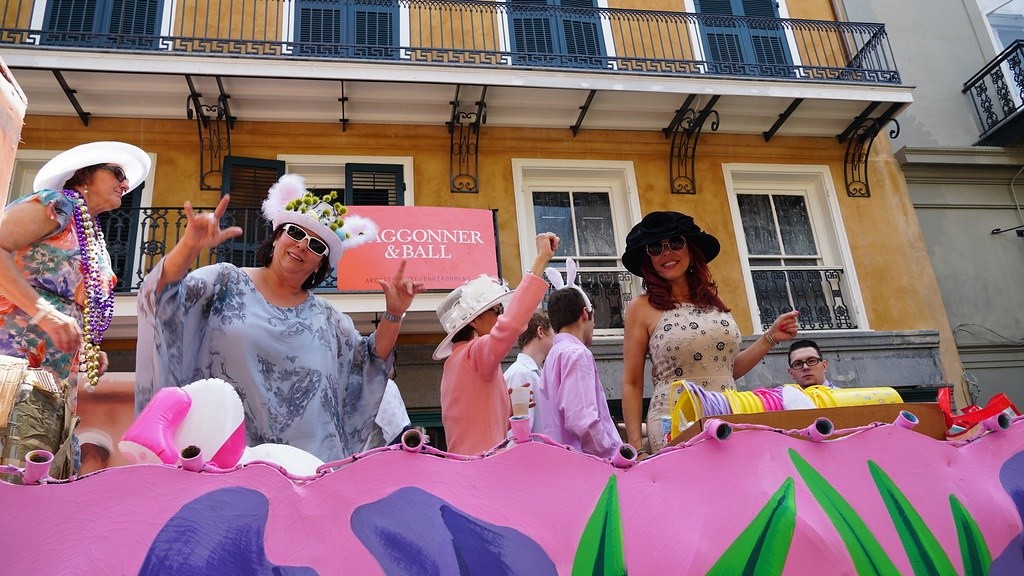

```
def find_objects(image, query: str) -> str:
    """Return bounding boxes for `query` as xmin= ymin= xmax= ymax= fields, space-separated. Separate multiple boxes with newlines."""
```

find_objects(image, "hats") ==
xmin=34 ymin=141 xmax=151 ymax=198
xmin=432 ymin=273 xmax=518 ymax=361
xmin=261 ymin=173 xmax=379 ymax=269
xmin=621 ymin=211 xmax=722 ymax=278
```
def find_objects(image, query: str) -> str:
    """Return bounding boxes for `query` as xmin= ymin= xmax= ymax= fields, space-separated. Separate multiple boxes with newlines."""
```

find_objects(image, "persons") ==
xmin=504 ymin=312 xmax=555 ymax=446
xmin=540 ymin=258 xmax=623 ymax=458
xmin=135 ymin=174 xmax=426 ymax=472
xmin=787 ymin=340 xmax=839 ymax=390
xmin=432 ymin=232 xmax=560 ymax=460
xmin=621 ymin=211 xmax=800 ymax=456
xmin=0 ymin=141 xmax=151 ymax=479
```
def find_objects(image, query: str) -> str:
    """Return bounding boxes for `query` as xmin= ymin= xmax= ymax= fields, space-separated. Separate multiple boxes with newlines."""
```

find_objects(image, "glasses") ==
xmin=282 ymin=223 xmax=330 ymax=260
xmin=645 ymin=235 xmax=690 ymax=256
xmin=488 ymin=306 xmax=504 ymax=317
xmin=93 ymin=164 xmax=130 ymax=193
xmin=789 ymin=357 xmax=823 ymax=370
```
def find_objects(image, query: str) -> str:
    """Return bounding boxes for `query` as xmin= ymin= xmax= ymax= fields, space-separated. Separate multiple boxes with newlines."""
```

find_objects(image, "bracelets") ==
xmin=637 ymin=447 xmax=648 ymax=455
xmin=384 ymin=311 xmax=406 ymax=322
xmin=28 ymin=303 xmax=55 ymax=325
xmin=764 ymin=329 xmax=779 ymax=346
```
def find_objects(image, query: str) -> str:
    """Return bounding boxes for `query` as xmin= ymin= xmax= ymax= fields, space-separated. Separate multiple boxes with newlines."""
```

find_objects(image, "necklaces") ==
xmin=63 ymin=188 xmax=114 ymax=393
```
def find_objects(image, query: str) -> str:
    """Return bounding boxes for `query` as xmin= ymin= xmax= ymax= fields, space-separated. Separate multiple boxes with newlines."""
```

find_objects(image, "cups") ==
xmin=508 ymin=386 xmax=531 ymax=416
